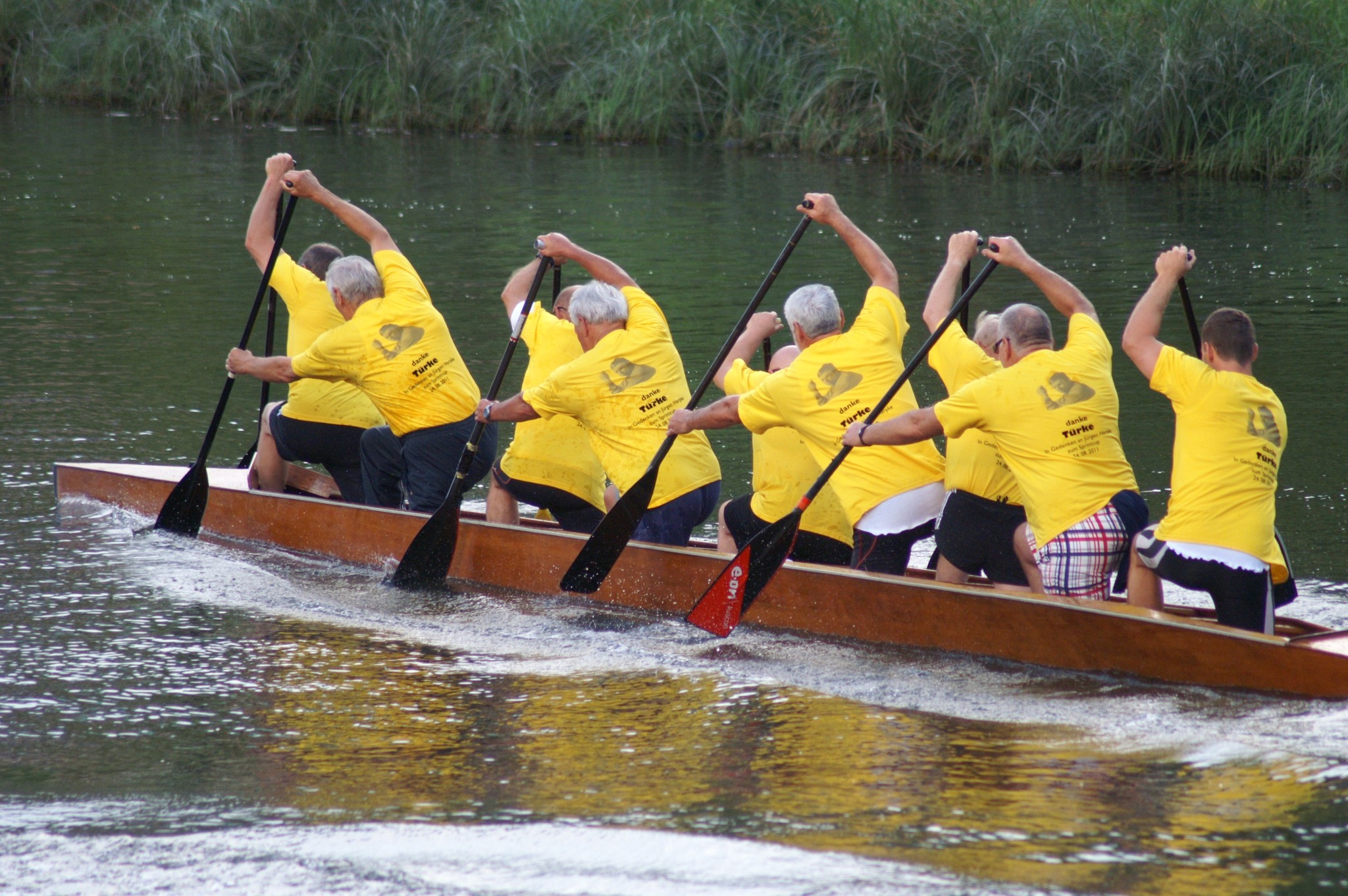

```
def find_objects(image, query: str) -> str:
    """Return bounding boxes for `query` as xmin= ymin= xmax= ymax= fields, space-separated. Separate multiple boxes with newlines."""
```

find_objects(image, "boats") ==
xmin=52 ymin=462 xmax=1348 ymax=700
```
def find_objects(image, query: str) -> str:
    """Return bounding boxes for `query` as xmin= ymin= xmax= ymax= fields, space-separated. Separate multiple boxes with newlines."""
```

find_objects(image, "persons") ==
xmin=1121 ymin=242 xmax=1289 ymax=639
xmin=599 ymin=357 xmax=656 ymax=394
xmin=223 ymin=150 xmax=1148 ymax=606
xmin=1038 ymin=373 xmax=1095 ymax=410
xmin=1247 ymin=404 xmax=1281 ymax=448
xmin=809 ymin=362 xmax=863 ymax=407
xmin=372 ymin=324 xmax=425 ymax=360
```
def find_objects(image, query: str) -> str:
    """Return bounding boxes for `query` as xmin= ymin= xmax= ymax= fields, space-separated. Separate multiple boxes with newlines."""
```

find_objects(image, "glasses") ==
xmin=992 ymin=337 xmax=1009 ymax=353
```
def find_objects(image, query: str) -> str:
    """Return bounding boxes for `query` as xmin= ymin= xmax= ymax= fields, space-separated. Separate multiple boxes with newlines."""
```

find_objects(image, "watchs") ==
xmin=857 ymin=424 xmax=874 ymax=447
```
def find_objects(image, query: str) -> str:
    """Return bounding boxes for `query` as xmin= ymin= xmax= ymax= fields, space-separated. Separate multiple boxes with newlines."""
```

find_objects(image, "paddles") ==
xmin=389 ymin=238 xmax=552 ymax=593
xmin=153 ymin=179 xmax=298 ymax=541
xmin=558 ymin=198 xmax=816 ymax=594
xmin=237 ymin=158 xmax=298 ymax=470
xmin=958 ymin=236 xmax=986 ymax=334
xmin=1178 ymin=252 xmax=1300 ymax=610
xmin=682 ymin=241 xmax=1000 ymax=640
xmin=535 ymin=263 xmax=561 ymax=519
